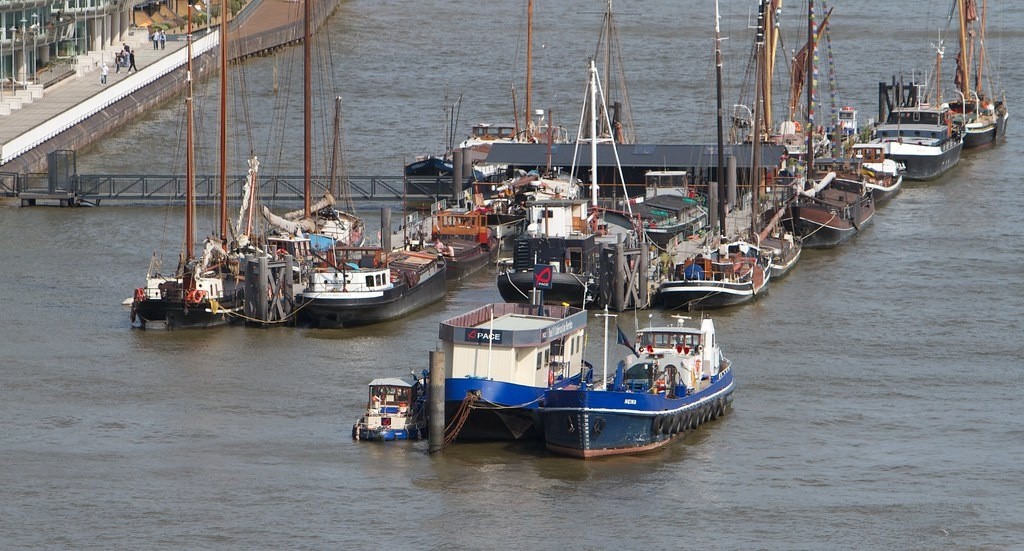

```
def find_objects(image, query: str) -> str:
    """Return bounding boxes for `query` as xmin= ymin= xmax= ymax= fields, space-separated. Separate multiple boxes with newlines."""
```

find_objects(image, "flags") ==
xmin=533 ymin=263 xmax=554 ymax=289
xmin=797 ymin=0 xmax=843 ymax=173
xmin=617 ymin=326 xmax=641 ymax=358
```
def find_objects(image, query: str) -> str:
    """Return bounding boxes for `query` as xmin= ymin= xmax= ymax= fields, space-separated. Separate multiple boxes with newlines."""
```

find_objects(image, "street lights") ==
xmin=0 ymin=27 xmax=12 ymax=116
xmin=4 ymin=26 xmax=22 ymax=110
xmin=15 ymin=17 xmax=34 ymax=104
xmin=25 ymin=13 xmax=44 ymax=99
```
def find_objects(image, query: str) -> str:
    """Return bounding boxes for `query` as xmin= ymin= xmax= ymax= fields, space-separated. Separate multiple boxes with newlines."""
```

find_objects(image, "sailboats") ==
xmin=122 ymin=0 xmax=1009 ymax=333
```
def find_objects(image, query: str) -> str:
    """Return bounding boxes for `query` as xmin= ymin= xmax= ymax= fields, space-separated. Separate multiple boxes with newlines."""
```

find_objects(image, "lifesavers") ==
xmin=793 ymin=121 xmax=801 ymax=132
xmin=192 ymin=290 xmax=203 ymax=303
xmin=942 ymin=112 xmax=952 ymax=122
xmin=549 ymin=369 xmax=554 ymax=386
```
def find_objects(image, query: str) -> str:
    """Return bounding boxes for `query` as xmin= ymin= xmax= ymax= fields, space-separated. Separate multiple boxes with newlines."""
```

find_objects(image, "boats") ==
xmin=351 ymin=370 xmax=429 ymax=442
xmin=439 ymin=264 xmax=594 ymax=442
xmin=539 ymin=304 xmax=736 ymax=460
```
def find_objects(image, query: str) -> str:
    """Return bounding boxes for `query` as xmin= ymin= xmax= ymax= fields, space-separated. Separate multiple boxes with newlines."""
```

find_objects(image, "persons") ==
xmin=152 ymin=29 xmax=159 ymax=50
xmin=126 ymin=50 xmax=139 ymax=73
xmin=100 ymin=63 xmax=108 ymax=86
xmin=159 ymin=30 xmax=166 ymax=49
xmin=115 ymin=43 xmax=130 ymax=74
